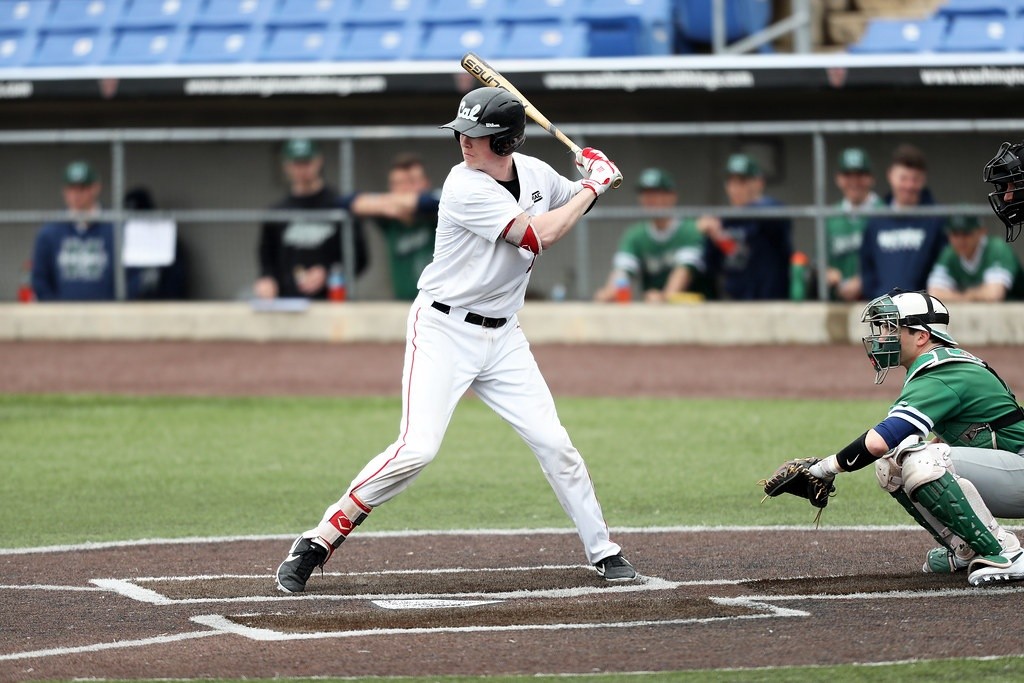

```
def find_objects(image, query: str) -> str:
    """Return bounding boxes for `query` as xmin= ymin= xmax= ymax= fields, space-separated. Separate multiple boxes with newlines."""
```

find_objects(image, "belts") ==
xmin=432 ymin=300 xmax=507 ymax=330
xmin=988 ymin=408 xmax=1024 ymax=431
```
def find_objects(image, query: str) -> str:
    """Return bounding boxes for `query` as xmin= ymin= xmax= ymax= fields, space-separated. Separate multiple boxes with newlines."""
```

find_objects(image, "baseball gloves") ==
xmin=762 ymin=456 xmax=836 ymax=509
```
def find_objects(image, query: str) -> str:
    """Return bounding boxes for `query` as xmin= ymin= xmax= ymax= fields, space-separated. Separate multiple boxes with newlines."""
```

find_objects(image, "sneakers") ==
xmin=275 ymin=536 xmax=327 ymax=594
xmin=968 ymin=549 xmax=1024 ymax=588
xmin=922 ymin=548 xmax=970 ymax=572
xmin=596 ymin=553 xmax=637 ymax=582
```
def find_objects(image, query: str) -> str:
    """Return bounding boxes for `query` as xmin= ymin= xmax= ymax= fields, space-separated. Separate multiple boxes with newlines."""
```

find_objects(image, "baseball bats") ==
xmin=459 ymin=51 xmax=625 ymax=190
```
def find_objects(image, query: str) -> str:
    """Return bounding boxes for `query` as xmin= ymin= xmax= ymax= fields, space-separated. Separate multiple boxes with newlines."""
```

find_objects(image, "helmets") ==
xmin=872 ymin=293 xmax=958 ymax=347
xmin=982 ymin=142 xmax=1024 ymax=243
xmin=438 ymin=87 xmax=525 ymax=157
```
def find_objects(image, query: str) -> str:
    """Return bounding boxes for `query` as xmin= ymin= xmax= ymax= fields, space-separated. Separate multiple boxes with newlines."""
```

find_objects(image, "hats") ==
xmin=944 ymin=216 xmax=982 ymax=238
xmin=278 ymin=139 xmax=316 ymax=164
xmin=64 ymin=163 xmax=96 ymax=186
xmin=837 ymin=147 xmax=871 ymax=173
xmin=723 ymin=155 xmax=762 ymax=179
xmin=636 ymin=168 xmax=680 ymax=189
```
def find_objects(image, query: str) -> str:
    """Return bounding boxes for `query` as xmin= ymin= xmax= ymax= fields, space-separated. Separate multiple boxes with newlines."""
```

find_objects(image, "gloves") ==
xmin=575 ymin=146 xmax=610 ymax=180
xmin=584 ymin=160 xmax=623 ymax=196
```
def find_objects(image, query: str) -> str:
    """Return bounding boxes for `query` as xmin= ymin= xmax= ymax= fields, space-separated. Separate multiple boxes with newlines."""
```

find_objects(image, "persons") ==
xmin=276 ymin=88 xmax=622 ymax=593
xmin=599 ymin=168 xmax=704 ymax=302
xmin=699 ymin=155 xmax=792 ymax=300
xmin=345 ymin=156 xmax=440 ymax=300
xmin=29 ymin=159 xmax=190 ymax=301
xmin=827 ymin=148 xmax=882 ymax=301
xmin=859 ymin=140 xmax=948 ymax=296
xmin=983 ymin=142 xmax=1024 ymax=243
xmin=927 ymin=216 xmax=1024 ymax=302
xmin=757 ymin=287 xmax=1024 ymax=585
xmin=255 ymin=138 xmax=371 ymax=299
